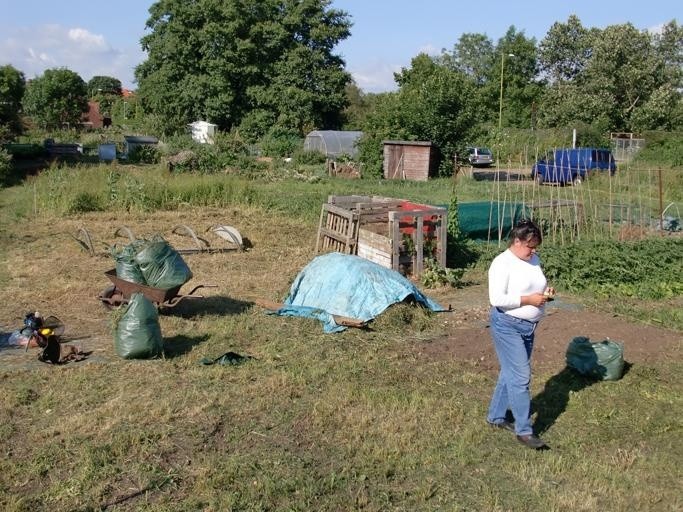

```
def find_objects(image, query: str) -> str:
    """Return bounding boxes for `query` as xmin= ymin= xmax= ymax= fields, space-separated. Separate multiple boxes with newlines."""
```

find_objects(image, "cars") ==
xmin=466 ymin=147 xmax=495 ymax=165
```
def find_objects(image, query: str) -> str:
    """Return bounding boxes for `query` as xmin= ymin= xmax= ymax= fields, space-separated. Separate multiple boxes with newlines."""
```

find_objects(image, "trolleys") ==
xmin=96 ymin=269 xmax=219 ymax=312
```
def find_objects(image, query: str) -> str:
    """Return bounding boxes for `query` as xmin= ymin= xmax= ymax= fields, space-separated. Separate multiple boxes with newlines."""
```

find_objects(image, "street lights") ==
xmin=495 ymin=51 xmax=515 ymax=168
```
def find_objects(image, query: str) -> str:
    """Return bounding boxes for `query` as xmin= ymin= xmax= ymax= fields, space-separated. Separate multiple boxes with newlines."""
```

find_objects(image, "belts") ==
xmin=495 ymin=306 xmax=539 ymax=324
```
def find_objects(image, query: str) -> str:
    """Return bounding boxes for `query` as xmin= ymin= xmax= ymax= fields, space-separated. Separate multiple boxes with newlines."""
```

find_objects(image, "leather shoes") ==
xmin=486 ymin=418 xmax=545 ymax=448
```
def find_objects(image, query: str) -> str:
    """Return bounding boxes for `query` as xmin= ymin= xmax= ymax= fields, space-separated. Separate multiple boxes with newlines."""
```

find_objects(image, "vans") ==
xmin=530 ymin=147 xmax=616 ymax=188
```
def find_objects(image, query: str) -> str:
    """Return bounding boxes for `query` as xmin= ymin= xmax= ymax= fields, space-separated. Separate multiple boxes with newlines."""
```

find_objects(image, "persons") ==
xmin=486 ymin=217 xmax=558 ymax=450
